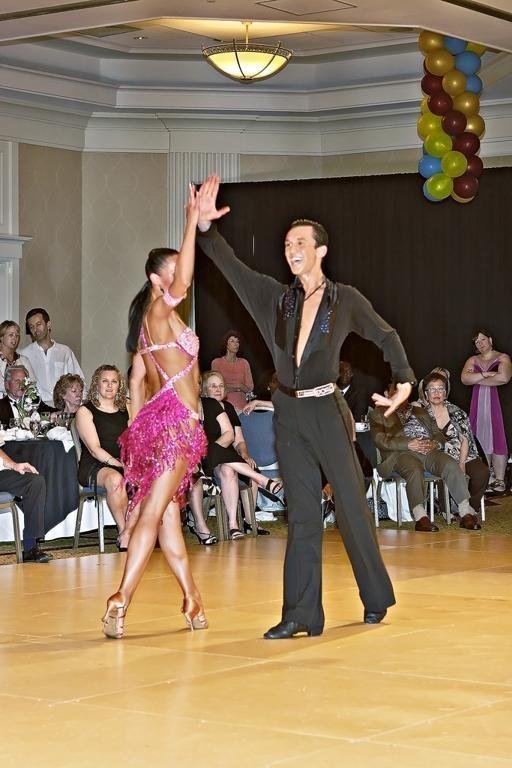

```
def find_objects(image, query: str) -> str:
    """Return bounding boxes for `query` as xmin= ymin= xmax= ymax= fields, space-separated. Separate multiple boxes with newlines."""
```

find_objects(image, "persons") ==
xmin=1 ymin=306 xmax=511 ymax=564
xmin=196 ymin=173 xmax=417 ymax=640
xmin=101 ymin=181 xmax=209 ymax=641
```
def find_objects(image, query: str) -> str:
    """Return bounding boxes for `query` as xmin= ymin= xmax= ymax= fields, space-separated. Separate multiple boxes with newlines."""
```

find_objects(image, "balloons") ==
xmin=417 ymin=28 xmax=486 ymax=206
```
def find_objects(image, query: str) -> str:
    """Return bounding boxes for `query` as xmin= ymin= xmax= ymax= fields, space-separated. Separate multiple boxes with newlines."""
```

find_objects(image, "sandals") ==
xmin=485 ymin=480 xmax=506 ymax=492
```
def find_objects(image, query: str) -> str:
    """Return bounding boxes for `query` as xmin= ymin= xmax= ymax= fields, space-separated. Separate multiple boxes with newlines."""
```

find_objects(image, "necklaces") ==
xmin=302 ymin=280 xmax=327 ymax=303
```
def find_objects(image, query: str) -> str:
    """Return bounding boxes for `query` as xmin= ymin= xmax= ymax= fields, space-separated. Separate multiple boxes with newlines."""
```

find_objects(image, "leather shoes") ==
xmin=22 ymin=546 xmax=53 ymax=562
xmin=459 ymin=514 xmax=481 ymax=530
xmin=364 ymin=608 xmax=386 ymax=623
xmin=265 ymin=622 xmax=322 ymax=639
xmin=415 ymin=517 xmax=438 ymax=532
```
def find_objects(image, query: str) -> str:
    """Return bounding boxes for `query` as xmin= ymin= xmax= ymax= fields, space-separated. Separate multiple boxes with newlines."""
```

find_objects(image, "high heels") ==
xmin=244 ymin=522 xmax=269 ymax=535
xmin=227 ymin=520 xmax=244 ymax=540
xmin=189 ymin=526 xmax=219 ymax=545
xmin=266 ymin=479 xmax=282 ymax=495
xmin=102 ymin=592 xmax=125 ymax=638
xmin=181 ymin=598 xmax=207 ymax=632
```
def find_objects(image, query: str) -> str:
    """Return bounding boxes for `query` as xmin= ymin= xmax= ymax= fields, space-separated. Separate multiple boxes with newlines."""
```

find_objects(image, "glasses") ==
xmin=424 ymin=386 xmax=447 ymax=393
xmin=209 ymin=384 xmax=227 ymax=391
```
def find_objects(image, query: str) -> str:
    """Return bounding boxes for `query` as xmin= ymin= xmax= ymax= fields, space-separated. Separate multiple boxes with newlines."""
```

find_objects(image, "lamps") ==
xmin=197 ymin=17 xmax=295 ymax=85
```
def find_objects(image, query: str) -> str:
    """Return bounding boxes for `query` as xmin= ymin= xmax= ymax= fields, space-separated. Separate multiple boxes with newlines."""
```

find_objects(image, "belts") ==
xmin=278 ymin=383 xmax=337 ymax=399
